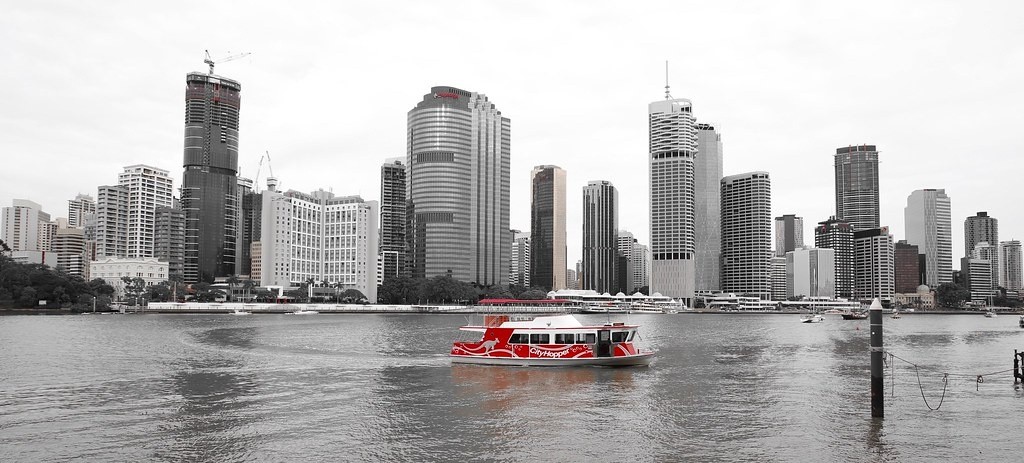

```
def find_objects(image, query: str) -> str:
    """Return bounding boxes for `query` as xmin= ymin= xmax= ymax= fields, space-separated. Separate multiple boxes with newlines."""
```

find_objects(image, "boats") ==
xmin=841 ymin=301 xmax=869 ymax=319
xmin=448 ymin=315 xmax=653 ymax=367
xmin=579 ymin=300 xmax=664 ymax=314
xmin=230 ymin=309 xmax=252 ymax=315
xmin=890 ymin=310 xmax=901 ymax=318
xmin=285 ymin=310 xmax=319 ymax=315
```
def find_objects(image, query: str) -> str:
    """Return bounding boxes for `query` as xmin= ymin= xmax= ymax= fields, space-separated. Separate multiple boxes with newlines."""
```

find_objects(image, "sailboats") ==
xmin=984 ymin=288 xmax=997 ymax=318
xmin=800 ymin=269 xmax=824 ymax=323
